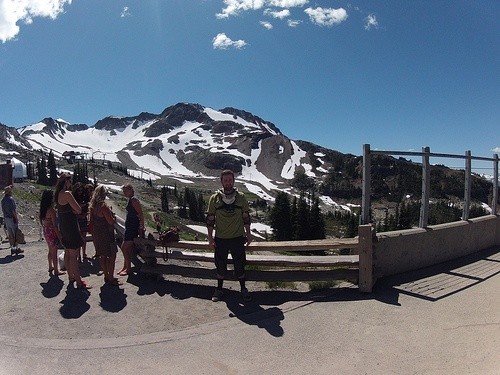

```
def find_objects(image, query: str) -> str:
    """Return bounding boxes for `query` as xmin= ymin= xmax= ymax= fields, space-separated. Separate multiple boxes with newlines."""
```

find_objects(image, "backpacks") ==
xmin=159 ymin=226 xmax=180 ymax=242
xmin=16 ymin=226 xmax=25 ymax=244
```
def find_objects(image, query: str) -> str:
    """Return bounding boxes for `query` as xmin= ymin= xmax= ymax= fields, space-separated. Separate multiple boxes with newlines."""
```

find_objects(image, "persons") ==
xmin=156 ymin=221 xmax=162 ymax=236
xmin=52 ymin=173 xmax=93 ymax=290
xmin=206 ymin=170 xmax=254 ymax=302
xmin=117 ymin=183 xmax=145 ymax=276
xmin=89 ymin=185 xmax=127 ymax=289
xmin=83 ymin=183 xmax=94 ymax=202
xmin=38 ymin=188 xmax=67 ymax=276
xmin=1 ymin=187 xmax=25 ymax=256
xmin=70 ymin=181 xmax=87 ymax=268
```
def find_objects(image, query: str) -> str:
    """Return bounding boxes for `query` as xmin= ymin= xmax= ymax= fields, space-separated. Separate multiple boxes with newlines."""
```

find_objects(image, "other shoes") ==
xmin=11 ymin=249 xmax=16 ymax=254
xmin=16 ymin=248 xmax=24 ymax=253
xmin=212 ymin=290 xmax=222 ymax=302
xmin=241 ymin=288 xmax=251 ymax=301
